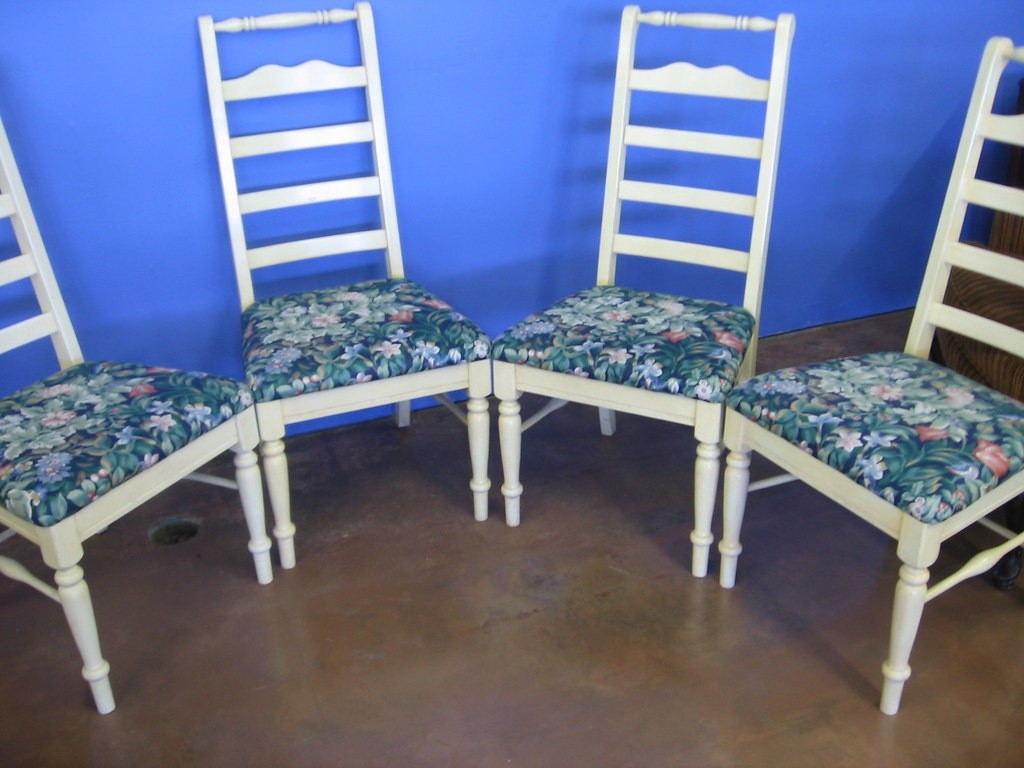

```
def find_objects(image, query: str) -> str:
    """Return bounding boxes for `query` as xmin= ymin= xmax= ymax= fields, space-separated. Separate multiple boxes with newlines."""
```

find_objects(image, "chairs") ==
xmin=717 ymin=36 xmax=1024 ymax=717
xmin=489 ymin=6 xmax=796 ymax=580
xmin=195 ymin=1 xmax=495 ymax=572
xmin=0 ymin=114 xmax=275 ymax=717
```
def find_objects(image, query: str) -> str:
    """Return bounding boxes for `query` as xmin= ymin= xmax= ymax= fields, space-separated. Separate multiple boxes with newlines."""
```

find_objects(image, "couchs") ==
xmin=928 ymin=210 xmax=1024 ymax=588
